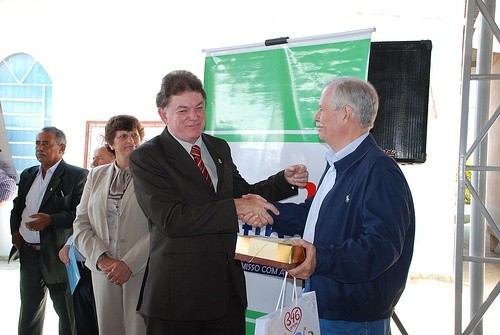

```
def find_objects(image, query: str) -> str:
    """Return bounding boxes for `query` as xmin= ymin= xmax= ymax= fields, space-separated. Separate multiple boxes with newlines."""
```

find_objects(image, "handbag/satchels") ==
xmin=255 ymin=270 xmax=320 ymax=335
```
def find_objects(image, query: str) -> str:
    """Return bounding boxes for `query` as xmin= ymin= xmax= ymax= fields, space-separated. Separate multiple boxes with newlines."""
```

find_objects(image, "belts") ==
xmin=25 ymin=243 xmax=41 ymax=251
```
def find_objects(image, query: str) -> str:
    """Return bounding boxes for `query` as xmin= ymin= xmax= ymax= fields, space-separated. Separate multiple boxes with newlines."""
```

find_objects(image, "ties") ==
xmin=191 ymin=144 xmax=217 ymax=194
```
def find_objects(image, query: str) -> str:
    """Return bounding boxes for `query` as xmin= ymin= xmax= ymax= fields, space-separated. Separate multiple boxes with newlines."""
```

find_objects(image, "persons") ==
xmin=236 ymin=77 xmax=415 ymax=335
xmin=59 ymin=146 xmax=114 ymax=335
xmin=73 ymin=114 xmax=150 ymax=335
xmin=11 ymin=126 xmax=90 ymax=335
xmin=128 ymin=71 xmax=308 ymax=335
